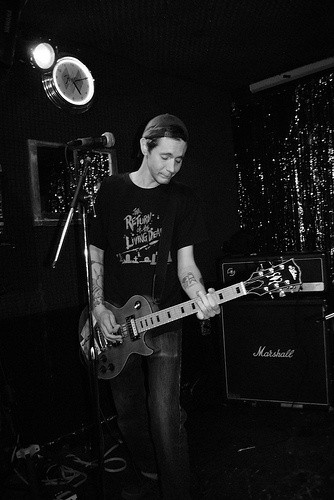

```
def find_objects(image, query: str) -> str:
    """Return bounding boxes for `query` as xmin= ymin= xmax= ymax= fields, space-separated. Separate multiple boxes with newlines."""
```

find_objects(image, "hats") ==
xmin=136 ymin=113 xmax=191 ymax=164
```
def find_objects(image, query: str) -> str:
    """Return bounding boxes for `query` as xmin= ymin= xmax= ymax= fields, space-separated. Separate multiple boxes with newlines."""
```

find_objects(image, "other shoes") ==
xmin=118 ymin=467 xmax=158 ymax=498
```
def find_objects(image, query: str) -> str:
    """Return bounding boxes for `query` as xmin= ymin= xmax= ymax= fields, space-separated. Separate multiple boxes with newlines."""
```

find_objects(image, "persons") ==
xmin=86 ymin=113 xmax=221 ymax=500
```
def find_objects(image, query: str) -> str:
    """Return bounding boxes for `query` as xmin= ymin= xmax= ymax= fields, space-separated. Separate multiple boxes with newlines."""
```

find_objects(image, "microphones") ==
xmin=67 ymin=131 xmax=116 ymax=149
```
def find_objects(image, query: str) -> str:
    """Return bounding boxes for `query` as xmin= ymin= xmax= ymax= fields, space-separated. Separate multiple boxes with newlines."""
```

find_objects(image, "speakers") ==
xmin=218 ymin=255 xmax=332 ymax=411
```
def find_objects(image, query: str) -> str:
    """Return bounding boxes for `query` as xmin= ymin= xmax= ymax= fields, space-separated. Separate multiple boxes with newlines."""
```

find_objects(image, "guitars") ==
xmin=79 ymin=255 xmax=305 ymax=380
